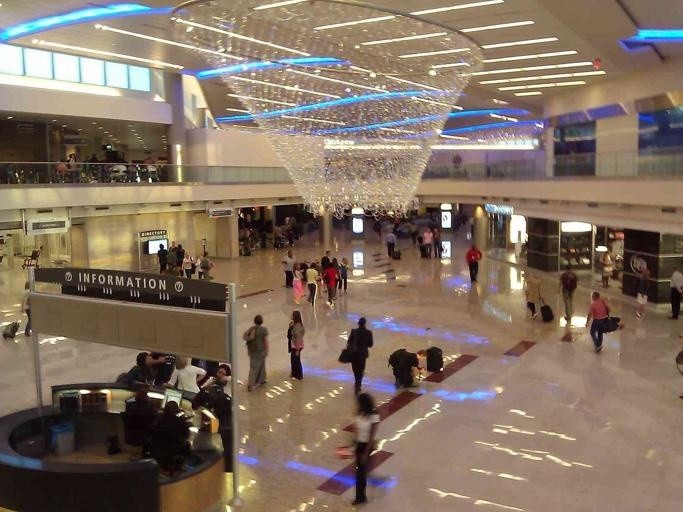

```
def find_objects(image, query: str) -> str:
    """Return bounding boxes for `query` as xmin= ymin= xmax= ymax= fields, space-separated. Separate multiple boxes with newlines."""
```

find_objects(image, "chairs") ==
xmin=124 ymin=401 xmax=190 ymax=463
xmin=0 ymin=163 xmax=166 ymax=186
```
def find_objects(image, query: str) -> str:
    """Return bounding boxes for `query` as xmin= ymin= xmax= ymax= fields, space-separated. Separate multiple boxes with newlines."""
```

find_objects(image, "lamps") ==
xmin=168 ymin=0 xmax=488 ymax=224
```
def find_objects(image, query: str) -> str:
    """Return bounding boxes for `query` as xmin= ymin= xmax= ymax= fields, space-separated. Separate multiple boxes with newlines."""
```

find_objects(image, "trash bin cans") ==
xmin=50 ymin=422 xmax=76 ymax=457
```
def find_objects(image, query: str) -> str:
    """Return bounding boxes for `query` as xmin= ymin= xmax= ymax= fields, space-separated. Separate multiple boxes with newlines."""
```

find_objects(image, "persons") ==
xmin=347 ymin=317 xmax=374 ymax=395
xmin=4 ymin=154 xmax=155 ymax=183
xmin=371 ymin=214 xmax=443 ymax=260
xmin=392 ymin=349 xmax=427 ymax=387
xmin=558 ymin=266 xmax=577 ymax=320
xmin=286 ymin=310 xmax=305 ymax=380
xmin=30 ymin=246 xmax=43 ymax=265
xmin=636 ymin=270 xmax=651 ymax=317
xmin=21 ymin=283 xmax=31 ymax=336
xmin=669 ymin=266 xmax=683 ymax=319
xmin=351 ymin=392 xmax=379 ymax=505
xmin=466 ymin=245 xmax=482 ymax=282
xmin=124 ymin=348 xmax=233 ymax=474
xmin=238 ymin=214 xmax=301 ymax=257
xmin=158 ymin=241 xmax=213 ymax=280
xmin=281 ymin=251 xmax=348 ymax=312
xmin=599 ymin=252 xmax=611 ymax=287
xmin=243 ymin=315 xmax=269 ymax=391
xmin=525 ymin=273 xmax=541 ymax=319
xmin=586 ymin=292 xmax=610 ymax=352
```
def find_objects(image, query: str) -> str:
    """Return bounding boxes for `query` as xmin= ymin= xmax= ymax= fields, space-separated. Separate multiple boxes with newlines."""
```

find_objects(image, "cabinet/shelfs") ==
xmin=559 ymin=231 xmax=591 ymax=270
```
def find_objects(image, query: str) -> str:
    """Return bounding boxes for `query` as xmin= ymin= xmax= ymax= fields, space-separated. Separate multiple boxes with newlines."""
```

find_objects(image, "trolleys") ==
xmin=21 ymin=245 xmax=42 ymax=270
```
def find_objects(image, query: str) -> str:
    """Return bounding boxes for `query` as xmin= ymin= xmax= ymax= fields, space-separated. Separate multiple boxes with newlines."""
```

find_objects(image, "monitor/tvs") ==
xmin=145 ymin=238 xmax=168 ymax=254
xmin=159 ymin=388 xmax=182 ymax=410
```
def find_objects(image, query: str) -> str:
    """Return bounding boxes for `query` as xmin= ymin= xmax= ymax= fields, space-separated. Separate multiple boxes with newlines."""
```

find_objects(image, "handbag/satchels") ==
xmin=339 ymin=350 xmax=352 ymax=363
xmin=605 ymin=316 xmax=620 ymax=332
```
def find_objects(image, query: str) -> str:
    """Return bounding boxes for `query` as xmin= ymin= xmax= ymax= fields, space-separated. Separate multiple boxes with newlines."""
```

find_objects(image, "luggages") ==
xmin=393 ymin=246 xmax=400 ymax=259
xmin=426 ymin=327 xmax=443 ymax=372
xmin=539 ymin=297 xmax=554 ymax=322
